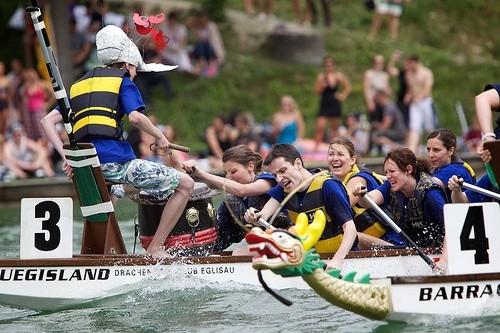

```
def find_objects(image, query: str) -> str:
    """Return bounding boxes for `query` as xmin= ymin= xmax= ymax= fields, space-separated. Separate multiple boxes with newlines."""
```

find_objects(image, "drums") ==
xmin=137 ymin=183 xmax=219 ymax=252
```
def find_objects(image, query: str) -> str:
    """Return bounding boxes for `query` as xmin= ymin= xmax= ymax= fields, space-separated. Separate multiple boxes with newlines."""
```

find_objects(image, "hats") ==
xmin=95 ymin=25 xmax=179 ymax=72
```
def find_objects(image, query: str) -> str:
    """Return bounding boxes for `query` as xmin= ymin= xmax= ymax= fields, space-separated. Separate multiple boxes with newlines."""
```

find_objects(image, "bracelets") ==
xmin=482 ymin=133 xmax=496 ymax=141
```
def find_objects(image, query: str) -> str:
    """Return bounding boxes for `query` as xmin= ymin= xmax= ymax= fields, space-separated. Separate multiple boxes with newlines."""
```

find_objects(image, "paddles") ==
xmin=360 ymin=185 xmax=446 ymax=275
xmin=254 ymin=209 xmax=343 ymax=279
xmin=458 ymin=175 xmax=500 ymax=200
xmin=26 ymin=6 xmax=109 ymax=223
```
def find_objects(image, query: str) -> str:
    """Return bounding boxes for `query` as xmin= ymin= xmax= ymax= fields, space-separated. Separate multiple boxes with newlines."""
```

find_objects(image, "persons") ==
xmin=272 ymin=95 xmax=305 ymax=145
xmin=182 ymin=145 xmax=291 ymax=253
xmin=205 ymin=110 xmax=260 ymax=160
xmin=70 ymin=0 xmax=224 ymax=99
xmin=387 ymin=52 xmax=439 ymax=150
xmin=364 ymin=54 xmax=392 ymax=154
xmin=326 ymin=136 xmax=385 ymax=238
xmin=371 ymin=0 xmax=403 ymax=42
xmin=475 ymin=84 xmax=500 ymax=163
xmin=338 ymin=113 xmax=368 ymax=152
xmin=314 ymin=57 xmax=352 ymax=152
xmin=371 ymin=89 xmax=405 ymax=150
xmin=243 ymin=0 xmax=331 ymax=28
xmin=353 ymin=148 xmax=449 ymax=273
xmin=447 ymin=174 xmax=500 ymax=205
xmin=425 ymin=129 xmax=476 ymax=202
xmin=244 ymin=144 xmax=359 ymax=270
xmin=0 ymin=58 xmax=69 ymax=182
xmin=39 ymin=24 xmax=194 ymax=258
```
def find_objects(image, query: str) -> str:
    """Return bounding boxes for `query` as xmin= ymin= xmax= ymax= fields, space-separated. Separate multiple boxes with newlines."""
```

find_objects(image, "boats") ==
xmin=245 ymin=202 xmax=500 ymax=323
xmin=0 ymin=141 xmax=489 ymax=311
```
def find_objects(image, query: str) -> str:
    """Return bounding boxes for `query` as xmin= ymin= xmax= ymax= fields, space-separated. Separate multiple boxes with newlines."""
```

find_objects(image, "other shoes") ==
xmin=367 ymin=146 xmax=380 ymax=157
xmin=163 ymin=89 xmax=177 ymax=102
xmin=195 ymin=61 xmax=216 ymax=77
xmin=381 ymin=144 xmax=391 ymax=156
